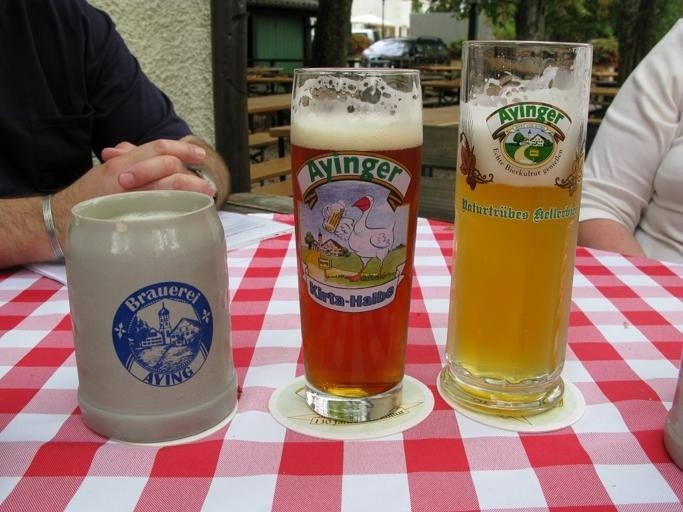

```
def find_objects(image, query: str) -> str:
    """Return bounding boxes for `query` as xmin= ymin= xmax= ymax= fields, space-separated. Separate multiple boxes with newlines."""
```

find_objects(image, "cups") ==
xmin=288 ymin=69 xmax=424 ymax=422
xmin=66 ymin=191 xmax=239 ymax=444
xmin=441 ymin=39 xmax=594 ymax=420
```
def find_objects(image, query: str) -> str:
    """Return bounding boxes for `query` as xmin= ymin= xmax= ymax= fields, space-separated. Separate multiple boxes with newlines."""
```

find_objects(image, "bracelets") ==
xmin=42 ymin=194 xmax=65 ymax=264
xmin=187 ymin=165 xmax=219 ymax=205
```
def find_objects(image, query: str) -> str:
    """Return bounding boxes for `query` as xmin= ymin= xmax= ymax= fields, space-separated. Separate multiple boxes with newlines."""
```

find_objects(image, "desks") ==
xmin=246 ymin=63 xmax=621 ymax=196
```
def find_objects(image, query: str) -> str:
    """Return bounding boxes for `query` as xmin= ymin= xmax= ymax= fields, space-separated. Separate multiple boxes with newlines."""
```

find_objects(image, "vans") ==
xmin=362 ymin=36 xmax=450 ymax=68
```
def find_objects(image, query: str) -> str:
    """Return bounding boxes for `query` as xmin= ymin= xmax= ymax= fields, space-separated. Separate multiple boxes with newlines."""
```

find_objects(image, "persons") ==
xmin=0 ymin=0 xmax=232 ymax=270
xmin=578 ymin=17 xmax=683 ymax=267
